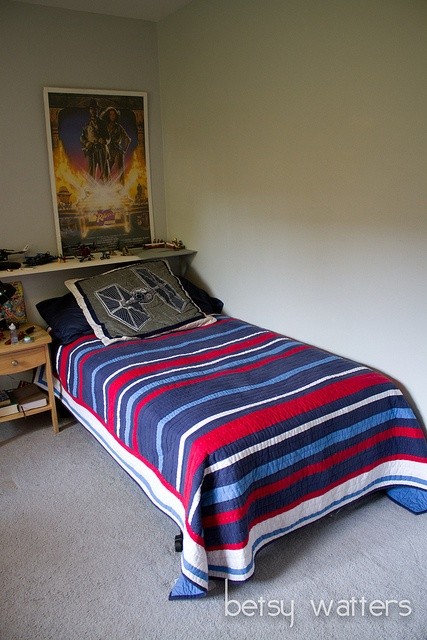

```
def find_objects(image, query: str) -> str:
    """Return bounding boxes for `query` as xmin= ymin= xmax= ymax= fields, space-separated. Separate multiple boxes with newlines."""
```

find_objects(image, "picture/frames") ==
xmin=41 ymin=83 xmax=156 ymax=256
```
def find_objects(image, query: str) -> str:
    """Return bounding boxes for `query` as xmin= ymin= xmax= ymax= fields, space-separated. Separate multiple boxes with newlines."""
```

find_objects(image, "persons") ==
xmin=97 ymin=107 xmax=131 ymax=185
xmin=80 ymin=98 xmax=111 ymax=185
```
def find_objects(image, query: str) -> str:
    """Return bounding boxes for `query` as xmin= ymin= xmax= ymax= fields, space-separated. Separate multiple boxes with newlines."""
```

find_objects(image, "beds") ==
xmin=32 ymin=273 xmax=427 ymax=599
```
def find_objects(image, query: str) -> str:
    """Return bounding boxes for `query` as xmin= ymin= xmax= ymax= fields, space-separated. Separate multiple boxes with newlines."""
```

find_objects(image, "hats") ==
xmin=87 ymin=99 xmax=101 ymax=109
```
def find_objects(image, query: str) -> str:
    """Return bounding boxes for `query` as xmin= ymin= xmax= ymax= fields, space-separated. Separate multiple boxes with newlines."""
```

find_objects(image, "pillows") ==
xmin=40 ymin=295 xmax=95 ymax=346
xmin=180 ymin=281 xmax=224 ymax=316
xmin=63 ymin=260 xmax=217 ymax=345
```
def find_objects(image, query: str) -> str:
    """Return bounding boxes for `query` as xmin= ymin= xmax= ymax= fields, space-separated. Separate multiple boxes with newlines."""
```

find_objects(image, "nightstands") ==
xmin=0 ymin=321 xmax=58 ymax=434
